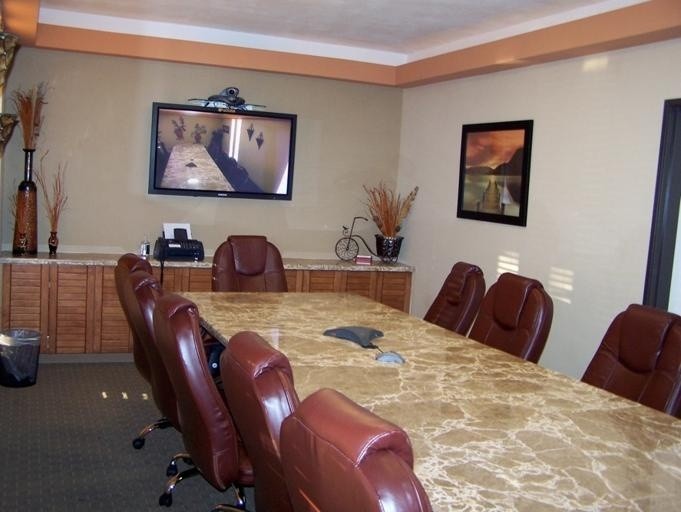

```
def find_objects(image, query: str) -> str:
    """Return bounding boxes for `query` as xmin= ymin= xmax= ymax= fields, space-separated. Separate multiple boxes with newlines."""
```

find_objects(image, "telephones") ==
xmin=153 ymin=236 xmax=165 ymax=258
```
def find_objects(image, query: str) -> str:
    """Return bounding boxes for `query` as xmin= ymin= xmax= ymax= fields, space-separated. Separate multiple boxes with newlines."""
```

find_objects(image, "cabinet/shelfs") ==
xmin=1 ymin=261 xmax=92 ymax=358
xmin=100 ymin=265 xmax=189 ymax=356
xmin=343 ymin=270 xmax=412 ymax=315
xmin=191 ymin=265 xmax=342 ymax=292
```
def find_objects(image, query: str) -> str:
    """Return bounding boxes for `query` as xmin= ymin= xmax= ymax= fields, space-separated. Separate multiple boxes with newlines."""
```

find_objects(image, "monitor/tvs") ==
xmin=147 ymin=101 xmax=298 ymax=200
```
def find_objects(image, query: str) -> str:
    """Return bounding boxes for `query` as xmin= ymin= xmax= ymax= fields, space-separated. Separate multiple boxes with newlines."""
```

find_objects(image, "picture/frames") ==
xmin=453 ymin=118 xmax=538 ymax=230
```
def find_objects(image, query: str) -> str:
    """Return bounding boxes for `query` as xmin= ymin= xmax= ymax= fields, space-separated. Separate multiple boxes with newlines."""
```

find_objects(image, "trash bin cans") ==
xmin=0 ymin=328 xmax=41 ymax=386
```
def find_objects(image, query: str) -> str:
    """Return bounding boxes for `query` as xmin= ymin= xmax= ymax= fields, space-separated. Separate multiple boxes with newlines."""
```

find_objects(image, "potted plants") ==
xmin=33 ymin=153 xmax=72 ymax=257
xmin=7 ymin=77 xmax=57 ymax=258
xmin=358 ymin=177 xmax=420 ymax=260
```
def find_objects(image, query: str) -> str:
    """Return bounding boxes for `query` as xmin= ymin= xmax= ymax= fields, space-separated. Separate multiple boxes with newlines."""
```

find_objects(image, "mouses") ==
xmin=376 ymin=351 xmax=404 ymax=363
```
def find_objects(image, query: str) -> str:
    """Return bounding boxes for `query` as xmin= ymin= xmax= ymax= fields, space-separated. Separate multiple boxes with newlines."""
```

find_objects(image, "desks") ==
xmin=160 ymin=279 xmax=681 ymax=512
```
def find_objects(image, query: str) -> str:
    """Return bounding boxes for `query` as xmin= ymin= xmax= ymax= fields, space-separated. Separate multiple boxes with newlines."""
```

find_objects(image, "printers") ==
xmin=153 ymin=230 xmax=205 ymax=261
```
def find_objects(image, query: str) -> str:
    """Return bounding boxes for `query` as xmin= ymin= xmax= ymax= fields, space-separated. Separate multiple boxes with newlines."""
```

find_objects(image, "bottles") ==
xmin=140 ymin=233 xmax=151 ymax=261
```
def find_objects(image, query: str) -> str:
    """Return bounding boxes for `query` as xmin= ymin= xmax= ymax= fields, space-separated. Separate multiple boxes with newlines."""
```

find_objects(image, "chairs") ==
xmin=468 ymin=268 xmax=556 ymax=366
xmin=219 ymin=328 xmax=301 ymax=512
xmin=111 ymin=252 xmax=155 ymax=283
xmin=150 ymin=285 xmax=260 ymax=512
xmin=277 ymin=386 xmax=435 ymax=511
xmin=423 ymin=258 xmax=489 ymax=338
xmin=582 ymin=301 xmax=680 ymax=422
xmin=209 ymin=234 xmax=291 ymax=295
xmin=107 ymin=273 xmax=217 ymax=453
